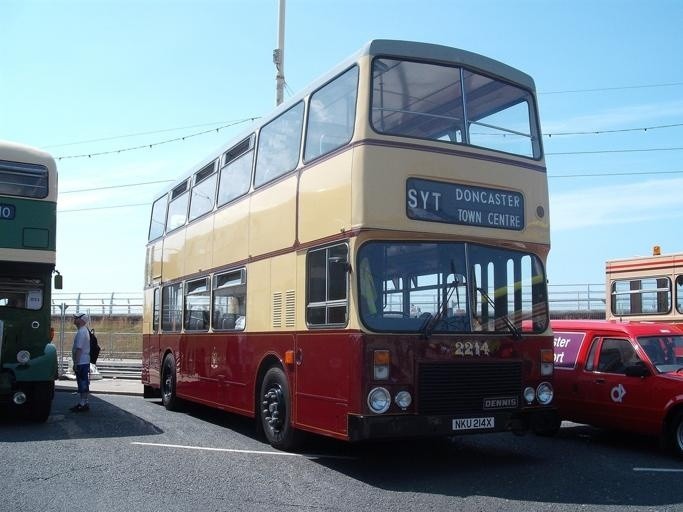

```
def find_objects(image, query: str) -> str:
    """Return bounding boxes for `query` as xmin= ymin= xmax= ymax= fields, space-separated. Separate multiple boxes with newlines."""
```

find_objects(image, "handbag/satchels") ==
xmin=86 ymin=328 xmax=100 ymax=363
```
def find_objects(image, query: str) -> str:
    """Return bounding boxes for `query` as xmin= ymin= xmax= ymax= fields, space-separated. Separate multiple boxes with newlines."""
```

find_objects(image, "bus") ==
xmin=138 ymin=36 xmax=567 ymax=457
xmin=0 ymin=139 xmax=63 ymax=431
xmin=599 ymin=244 xmax=683 ymax=321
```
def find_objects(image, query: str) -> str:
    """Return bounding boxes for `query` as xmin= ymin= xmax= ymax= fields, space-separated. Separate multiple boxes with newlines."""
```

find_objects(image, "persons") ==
xmin=70 ymin=313 xmax=90 ymax=412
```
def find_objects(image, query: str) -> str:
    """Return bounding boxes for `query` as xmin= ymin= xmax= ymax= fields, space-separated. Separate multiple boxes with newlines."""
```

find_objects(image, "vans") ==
xmin=521 ymin=316 xmax=683 ymax=457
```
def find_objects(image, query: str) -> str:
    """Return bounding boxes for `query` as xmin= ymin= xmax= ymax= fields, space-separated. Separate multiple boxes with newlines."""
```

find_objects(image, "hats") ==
xmin=73 ymin=312 xmax=89 ymax=323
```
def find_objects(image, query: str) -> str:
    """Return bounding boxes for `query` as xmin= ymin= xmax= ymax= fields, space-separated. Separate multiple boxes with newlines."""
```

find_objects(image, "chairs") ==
xmin=189 ymin=315 xmax=240 ymax=330
xmin=606 ymin=350 xmax=626 ymax=373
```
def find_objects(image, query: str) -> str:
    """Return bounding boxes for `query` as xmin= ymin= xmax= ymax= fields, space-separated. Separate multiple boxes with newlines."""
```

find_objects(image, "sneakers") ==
xmin=71 ymin=403 xmax=89 ymax=410
